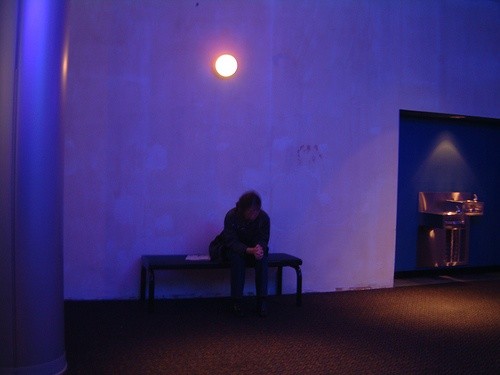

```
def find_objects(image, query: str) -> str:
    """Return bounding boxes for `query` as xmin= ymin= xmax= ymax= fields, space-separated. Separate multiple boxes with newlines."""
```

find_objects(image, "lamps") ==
xmin=211 ymin=50 xmax=240 ymax=81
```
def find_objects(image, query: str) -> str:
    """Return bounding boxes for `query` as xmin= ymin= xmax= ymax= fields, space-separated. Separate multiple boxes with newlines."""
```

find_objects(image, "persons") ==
xmin=208 ymin=190 xmax=273 ymax=314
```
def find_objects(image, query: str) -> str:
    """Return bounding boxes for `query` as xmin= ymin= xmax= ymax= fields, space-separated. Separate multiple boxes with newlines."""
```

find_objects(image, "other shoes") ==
xmin=255 ymin=304 xmax=270 ymax=317
xmin=232 ymin=302 xmax=247 ymax=317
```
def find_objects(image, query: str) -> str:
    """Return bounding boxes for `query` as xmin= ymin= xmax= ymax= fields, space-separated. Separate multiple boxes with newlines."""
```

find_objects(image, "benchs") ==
xmin=139 ymin=252 xmax=303 ymax=312
